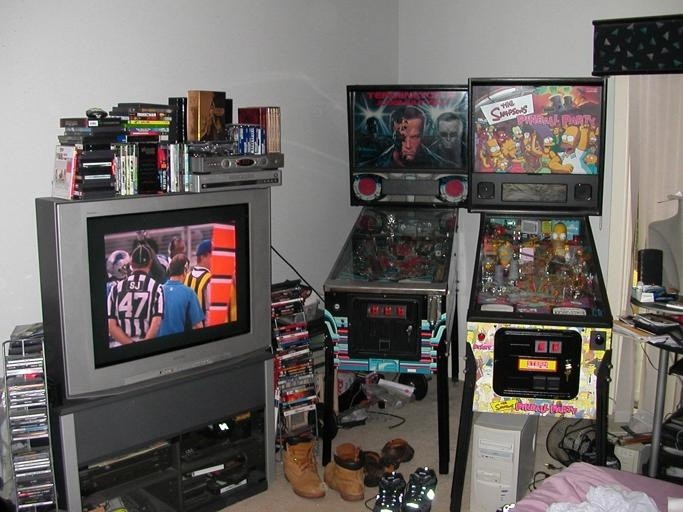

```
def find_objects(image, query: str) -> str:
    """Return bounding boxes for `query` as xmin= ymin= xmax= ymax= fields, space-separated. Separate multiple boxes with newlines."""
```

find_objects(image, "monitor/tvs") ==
xmin=35 ymin=184 xmax=274 ymax=406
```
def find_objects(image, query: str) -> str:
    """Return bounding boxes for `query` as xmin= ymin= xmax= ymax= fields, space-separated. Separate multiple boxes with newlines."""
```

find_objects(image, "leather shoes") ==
xmin=360 ymin=451 xmax=380 ymax=486
xmin=380 ymin=439 xmax=415 ymax=470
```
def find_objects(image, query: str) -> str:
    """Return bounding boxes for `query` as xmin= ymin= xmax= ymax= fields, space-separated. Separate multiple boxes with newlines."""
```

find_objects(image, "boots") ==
xmin=283 ymin=436 xmax=325 ymax=498
xmin=324 ymin=443 xmax=365 ymax=501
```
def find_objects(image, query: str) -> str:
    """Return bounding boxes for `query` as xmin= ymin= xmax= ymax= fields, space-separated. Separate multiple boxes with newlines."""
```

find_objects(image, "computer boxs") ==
xmin=470 ymin=414 xmax=539 ymax=512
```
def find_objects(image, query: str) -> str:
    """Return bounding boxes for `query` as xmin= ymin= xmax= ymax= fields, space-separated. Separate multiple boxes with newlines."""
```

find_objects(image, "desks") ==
xmin=613 ymin=315 xmax=683 ymax=487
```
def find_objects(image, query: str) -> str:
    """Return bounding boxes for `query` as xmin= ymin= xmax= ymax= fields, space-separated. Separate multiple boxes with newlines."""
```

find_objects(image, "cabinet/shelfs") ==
xmin=448 ymin=211 xmax=611 ymax=512
xmin=321 ymin=203 xmax=458 ymax=475
xmin=55 ymin=348 xmax=275 ymax=512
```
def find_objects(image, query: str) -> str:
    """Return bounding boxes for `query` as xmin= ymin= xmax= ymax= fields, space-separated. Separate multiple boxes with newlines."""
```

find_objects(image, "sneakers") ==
xmin=372 ymin=472 xmax=406 ymax=512
xmin=402 ymin=466 xmax=437 ymax=511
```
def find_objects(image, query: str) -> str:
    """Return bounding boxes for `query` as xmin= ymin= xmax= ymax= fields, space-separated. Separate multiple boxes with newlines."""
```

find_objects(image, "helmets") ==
xmin=106 ymin=250 xmax=131 ymax=275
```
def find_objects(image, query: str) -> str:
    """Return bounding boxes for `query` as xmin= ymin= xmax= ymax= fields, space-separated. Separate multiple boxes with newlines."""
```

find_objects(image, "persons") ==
xmin=167 ymin=237 xmax=185 ymax=263
xmin=184 ymin=240 xmax=212 ymax=328
xmin=157 ymin=255 xmax=205 ymax=338
xmin=427 ymin=112 xmax=469 ymax=168
xmin=106 ymin=250 xmax=127 ymax=297
xmin=107 ymin=245 xmax=164 ymax=348
xmin=132 ymin=230 xmax=159 ymax=255
xmin=357 ymin=107 xmax=459 ymax=169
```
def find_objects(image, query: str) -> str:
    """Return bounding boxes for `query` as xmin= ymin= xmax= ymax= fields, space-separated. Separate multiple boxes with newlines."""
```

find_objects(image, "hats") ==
xmin=196 ymin=240 xmax=211 ymax=254
xmin=168 ymin=254 xmax=190 ymax=275
xmin=130 ymin=245 xmax=151 ymax=268
